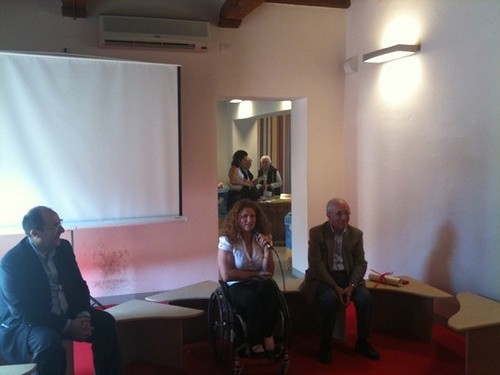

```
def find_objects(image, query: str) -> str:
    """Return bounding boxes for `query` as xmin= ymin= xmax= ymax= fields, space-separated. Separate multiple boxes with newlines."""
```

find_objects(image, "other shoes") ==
xmin=355 ymin=340 xmax=380 ymax=360
xmin=319 ymin=342 xmax=332 ymax=363
xmin=251 ymin=345 xmax=281 ymax=360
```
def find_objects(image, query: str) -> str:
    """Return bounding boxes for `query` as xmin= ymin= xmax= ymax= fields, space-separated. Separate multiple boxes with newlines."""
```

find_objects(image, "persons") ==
xmin=218 ymin=198 xmax=282 ymax=361
xmin=0 ymin=204 xmax=123 ymax=375
xmin=298 ymin=197 xmax=383 ymax=365
xmin=226 ymin=149 xmax=283 ymax=210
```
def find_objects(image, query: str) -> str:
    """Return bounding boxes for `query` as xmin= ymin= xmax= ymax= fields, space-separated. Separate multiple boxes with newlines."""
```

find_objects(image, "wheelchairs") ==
xmin=208 ymin=279 xmax=291 ymax=375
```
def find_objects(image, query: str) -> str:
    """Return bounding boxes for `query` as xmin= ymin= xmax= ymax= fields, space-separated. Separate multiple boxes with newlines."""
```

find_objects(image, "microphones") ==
xmin=253 ymin=230 xmax=272 ymax=248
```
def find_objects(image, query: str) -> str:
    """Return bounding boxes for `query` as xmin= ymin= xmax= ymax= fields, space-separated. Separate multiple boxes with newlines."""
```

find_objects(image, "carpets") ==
xmin=74 ymin=300 xmax=466 ymax=375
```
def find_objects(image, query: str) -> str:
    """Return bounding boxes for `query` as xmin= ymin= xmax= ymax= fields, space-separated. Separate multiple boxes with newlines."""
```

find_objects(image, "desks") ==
xmin=256 ymin=197 xmax=291 ymax=247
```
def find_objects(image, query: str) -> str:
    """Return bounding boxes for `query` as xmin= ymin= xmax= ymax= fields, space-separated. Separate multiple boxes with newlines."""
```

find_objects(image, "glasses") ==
xmin=53 ymin=221 xmax=62 ymax=232
xmin=335 ymin=211 xmax=351 ymax=217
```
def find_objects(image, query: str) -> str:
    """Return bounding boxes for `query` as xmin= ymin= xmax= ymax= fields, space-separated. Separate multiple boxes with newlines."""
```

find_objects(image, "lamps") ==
xmin=363 ymin=45 xmax=418 ymax=63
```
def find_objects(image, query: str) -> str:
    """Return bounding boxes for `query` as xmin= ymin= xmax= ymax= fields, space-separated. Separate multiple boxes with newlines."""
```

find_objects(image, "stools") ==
xmin=145 ymin=280 xmax=223 ymax=343
xmin=104 ymin=299 xmax=205 ymax=370
xmin=278 ymin=278 xmax=345 ymax=340
xmin=0 ymin=336 xmax=74 ymax=375
xmin=447 ymin=291 xmax=500 ymax=375
xmin=365 ymin=274 xmax=454 ymax=343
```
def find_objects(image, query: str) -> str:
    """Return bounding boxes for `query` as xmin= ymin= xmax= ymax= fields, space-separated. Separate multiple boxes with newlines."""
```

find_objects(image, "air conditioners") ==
xmin=98 ymin=15 xmax=210 ymax=53
